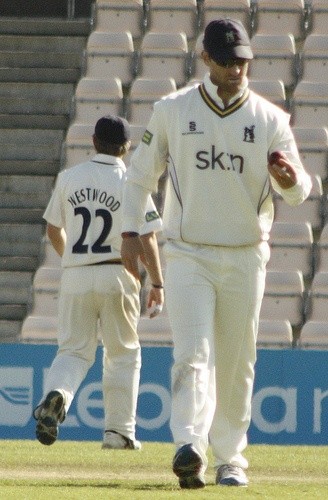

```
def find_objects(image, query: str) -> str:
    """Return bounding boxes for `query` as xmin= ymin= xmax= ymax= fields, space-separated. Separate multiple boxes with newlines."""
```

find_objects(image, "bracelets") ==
xmin=152 ymin=284 xmax=163 ymax=289
xmin=121 ymin=232 xmax=140 ymax=239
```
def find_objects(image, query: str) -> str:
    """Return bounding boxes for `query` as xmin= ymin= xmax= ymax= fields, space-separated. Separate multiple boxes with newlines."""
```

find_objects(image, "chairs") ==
xmin=21 ymin=0 xmax=328 ymax=353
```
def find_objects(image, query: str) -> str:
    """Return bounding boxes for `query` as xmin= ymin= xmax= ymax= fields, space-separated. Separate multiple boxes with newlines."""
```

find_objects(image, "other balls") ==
xmin=268 ymin=152 xmax=287 ymax=170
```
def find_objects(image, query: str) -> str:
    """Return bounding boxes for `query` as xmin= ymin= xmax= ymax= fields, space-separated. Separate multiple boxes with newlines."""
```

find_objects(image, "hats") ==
xmin=205 ymin=18 xmax=254 ymax=60
xmin=95 ymin=115 xmax=131 ymax=140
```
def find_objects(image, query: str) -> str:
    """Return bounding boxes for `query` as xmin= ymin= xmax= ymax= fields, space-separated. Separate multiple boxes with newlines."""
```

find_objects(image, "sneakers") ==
xmin=35 ymin=390 xmax=67 ymax=445
xmin=101 ymin=431 xmax=142 ymax=450
xmin=216 ymin=465 xmax=250 ymax=486
xmin=172 ymin=443 xmax=207 ymax=489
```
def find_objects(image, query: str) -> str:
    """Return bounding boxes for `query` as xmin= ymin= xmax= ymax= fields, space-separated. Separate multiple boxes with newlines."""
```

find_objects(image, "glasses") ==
xmin=215 ymin=59 xmax=247 ymax=68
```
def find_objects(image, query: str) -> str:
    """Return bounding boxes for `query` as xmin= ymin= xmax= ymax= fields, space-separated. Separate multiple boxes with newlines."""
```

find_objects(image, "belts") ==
xmin=90 ymin=261 xmax=123 ymax=265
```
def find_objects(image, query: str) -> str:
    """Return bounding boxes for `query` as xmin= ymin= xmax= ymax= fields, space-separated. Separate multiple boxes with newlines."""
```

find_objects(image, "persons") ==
xmin=34 ymin=116 xmax=165 ymax=449
xmin=121 ymin=20 xmax=313 ymax=489
